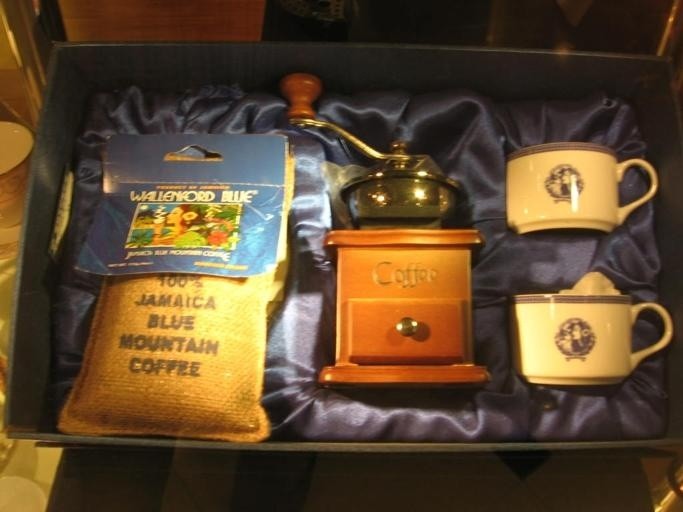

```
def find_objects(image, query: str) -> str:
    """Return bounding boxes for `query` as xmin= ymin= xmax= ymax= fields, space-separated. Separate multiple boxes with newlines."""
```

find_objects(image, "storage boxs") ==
xmin=3 ymin=38 xmax=682 ymax=457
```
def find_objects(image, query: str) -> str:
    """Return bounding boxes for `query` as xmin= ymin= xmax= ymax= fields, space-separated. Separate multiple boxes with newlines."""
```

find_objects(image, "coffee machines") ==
xmin=281 ymin=69 xmax=492 ymax=395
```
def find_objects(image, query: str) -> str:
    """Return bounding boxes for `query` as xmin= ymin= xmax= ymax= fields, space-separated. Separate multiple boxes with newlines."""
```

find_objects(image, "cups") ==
xmin=508 ymin=294 xmax=674 ymax=386
xmin=0 ymin=120 xmax=33 ymax=229
xmin=505 ymin=142 xmax=659 ymax=239
